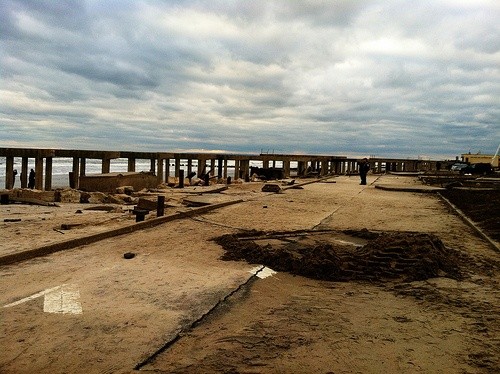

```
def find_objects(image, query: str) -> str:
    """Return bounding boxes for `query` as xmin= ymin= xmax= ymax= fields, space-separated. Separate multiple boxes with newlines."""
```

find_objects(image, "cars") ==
xmin=451 ymin=163 xmax=495 ymax=176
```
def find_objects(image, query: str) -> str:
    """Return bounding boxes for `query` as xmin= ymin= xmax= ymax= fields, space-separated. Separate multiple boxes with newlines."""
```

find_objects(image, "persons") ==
xmin=357 ymin=158 xmax=369 ymax=185
xmin=28 ymin=169 xmax=35 ymax=189
xmin=12 ymin=169 xmax=17 ymax=188
